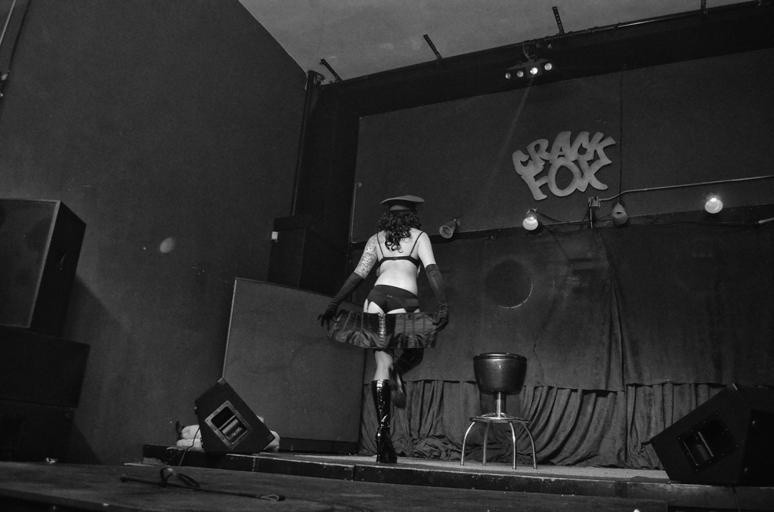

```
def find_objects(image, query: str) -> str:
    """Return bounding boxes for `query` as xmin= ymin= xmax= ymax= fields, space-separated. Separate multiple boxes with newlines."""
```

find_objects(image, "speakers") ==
xmin=649 ymin=383 xmax=774 ymax=486
xmin=0 ymin=199 xmax=86 ymax=330
xmin=0 ymin=401 xmax=76 ymax=462
xmin=0 ymin=328 xmax=90 ymax=409
xmin=194 ymin=378 xmax=276 ymax=454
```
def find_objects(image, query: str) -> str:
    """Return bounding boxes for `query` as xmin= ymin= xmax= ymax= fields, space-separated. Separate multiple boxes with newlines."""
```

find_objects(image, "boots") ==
xmin=371 ymin=378 xmax=397 ymax=463
xmin=388 ymin=347 xmax=424 ymax=410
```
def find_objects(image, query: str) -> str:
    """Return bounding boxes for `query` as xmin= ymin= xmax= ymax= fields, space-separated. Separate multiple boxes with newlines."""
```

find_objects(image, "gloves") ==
xmin=317 ymin=271 xmax=364 ymax=330
xmin=423 ymin=263 xmax=449 ymax=333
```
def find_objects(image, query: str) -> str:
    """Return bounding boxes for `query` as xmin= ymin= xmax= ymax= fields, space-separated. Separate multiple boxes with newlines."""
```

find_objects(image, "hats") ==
xmin=380 ymin=194 xmax=425 ymax=212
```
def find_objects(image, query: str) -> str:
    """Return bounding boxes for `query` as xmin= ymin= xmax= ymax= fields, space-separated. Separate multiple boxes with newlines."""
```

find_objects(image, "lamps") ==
xmin=703 ymin=191 xmax=724 ymax=214
xmin=521 ymin=206 xmax=562 ymax=232
xmin=439 ymin=218 xmax=457 ymax=239
xmin=503 ymin=57 xmax=554 ymax=80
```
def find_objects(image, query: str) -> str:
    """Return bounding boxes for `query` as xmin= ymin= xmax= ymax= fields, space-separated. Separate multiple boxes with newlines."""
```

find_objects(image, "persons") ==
xmin=318 ymin=194 xmax=450 ymax=465
